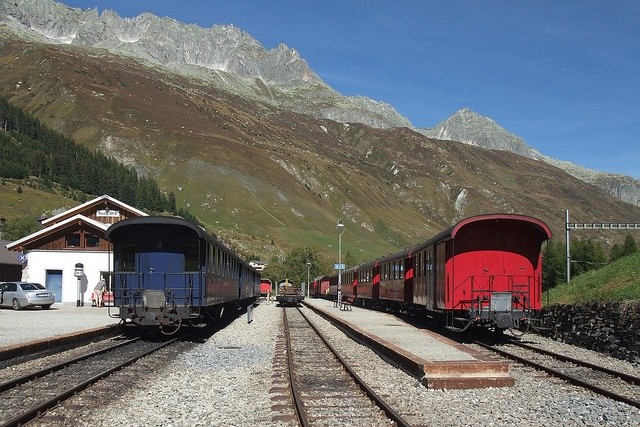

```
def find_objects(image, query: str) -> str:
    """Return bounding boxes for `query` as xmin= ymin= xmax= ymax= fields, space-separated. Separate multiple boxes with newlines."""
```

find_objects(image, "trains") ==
xmin=275 ymin=278 xmax=306 ymax=309
xmin=307 ymin=212 xmax=553 ymax=336
xmin=104 ymin=214 xmax=273 ymax=335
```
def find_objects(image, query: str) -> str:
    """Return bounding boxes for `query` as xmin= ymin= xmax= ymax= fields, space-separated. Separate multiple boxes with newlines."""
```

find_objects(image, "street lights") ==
xmin=306 ymin=259 xmax=312 ymax=297
xmin=335 ymin=218 xmax=346 ymax=308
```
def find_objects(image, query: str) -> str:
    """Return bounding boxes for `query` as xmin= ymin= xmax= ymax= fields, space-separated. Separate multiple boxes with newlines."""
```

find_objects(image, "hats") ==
xmin=102 ymin=279 xmax=105 ymax=281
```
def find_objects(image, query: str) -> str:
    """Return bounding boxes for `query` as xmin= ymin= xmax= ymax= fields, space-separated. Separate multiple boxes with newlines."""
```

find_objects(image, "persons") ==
xmin=94 ymin=279 xmax=108 ymax=307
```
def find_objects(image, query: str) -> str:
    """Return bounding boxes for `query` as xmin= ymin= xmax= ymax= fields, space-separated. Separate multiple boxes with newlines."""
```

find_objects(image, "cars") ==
xmin=0 ymin=280 xmax=56 ymax=310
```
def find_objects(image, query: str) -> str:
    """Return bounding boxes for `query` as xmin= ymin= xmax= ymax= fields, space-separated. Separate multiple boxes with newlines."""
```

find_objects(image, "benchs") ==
xmin=333 ymin=295 xmax=344 ymax=308
xmin=340 ymin=294 xmax=355 ymax=311
